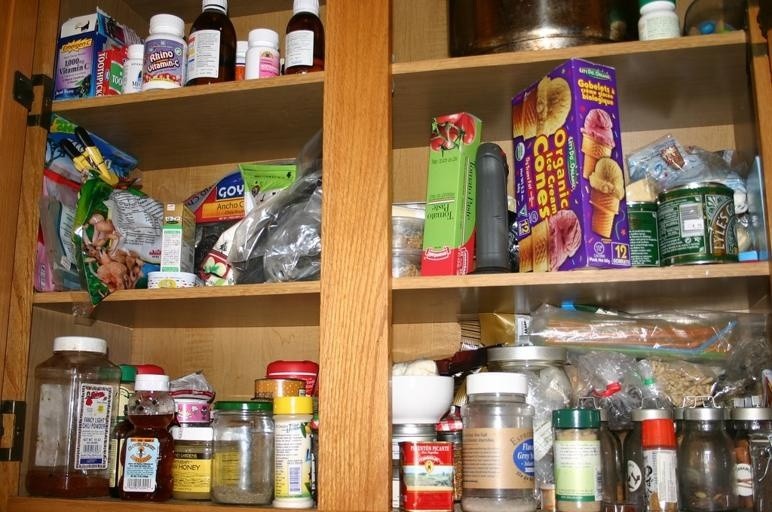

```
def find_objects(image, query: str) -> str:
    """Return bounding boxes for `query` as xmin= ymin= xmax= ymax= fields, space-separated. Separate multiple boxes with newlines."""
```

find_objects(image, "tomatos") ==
xmin=454 ymin=115 xmax=476 ymax=144
xmin=436 ymin=112 xmax=464 ymax=127
xmin=431 ymin=126 xmax=459 ymax=152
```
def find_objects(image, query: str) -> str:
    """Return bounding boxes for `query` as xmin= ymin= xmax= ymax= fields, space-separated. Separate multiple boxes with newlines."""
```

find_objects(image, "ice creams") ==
xmin=517 ymin=209 xmax=581 ymax=273
xmin=582 ymin=108 xmax=617 ymax=179
xmin=511 ymin=76 xmax=571 ymax=140
xmin=588 ymin=158 xmax=625 ymax=239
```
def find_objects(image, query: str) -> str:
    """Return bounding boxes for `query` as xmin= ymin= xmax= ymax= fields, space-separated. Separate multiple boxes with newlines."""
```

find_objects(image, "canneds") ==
xmin=656 ymin=181 xmax=739 ymax=266
xmin=624 ymin=200 xmax=659 ymax=267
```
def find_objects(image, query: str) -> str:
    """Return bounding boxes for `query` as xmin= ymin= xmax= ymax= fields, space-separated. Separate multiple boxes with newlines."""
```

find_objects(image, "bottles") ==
xmin=123 ymin=44 xmax=144 ymax=93
xmin=235 ymin=40 xmax=248 ymax=79
xmin=436 ymin=420 xmax=462 ymax=503
xmin=474 ymin=141 xmax=510 ymax=272
xmin=284 ymin=0 xmax=324 ymax=73
xmin=597 ymin=410 xmax=624 ymax=506
xmin=730 ymin=407 xmax=772 ymax=509
xmin=622 ymin=409 xmax=670 ymax=504
xmin=25 ymin=336 xmax=122 ymax=499
xmin=553 ymin=408 xmax=602 ymax=512
xmin=640 ymin=419 xmax=680 ymax=511
xmin=244 ymin=27 xmax=280 ymax=80
xmin=184 ymin=0 xmax=237 ymax=86
xmin=680 ymin=408 xmax=730 ymax=512
xmin=272 ymin=396 xmax=313 ymax=508
xmin=486 ymin=346 xmax=572 ymax=509
xmin=119 ymin=373 xmax=175 ymax=502
xmin=637 ymin=0 xmax=680 ymax=41
xmin=673 ymin=409 xmax=685 ymax=444
xmin=460 ymin=372 xmax=537 ymax=512
xmin=110 ymin=364 xmax=137 ymax=500
xmin=142 ymin=14 xmax=187 ymax=92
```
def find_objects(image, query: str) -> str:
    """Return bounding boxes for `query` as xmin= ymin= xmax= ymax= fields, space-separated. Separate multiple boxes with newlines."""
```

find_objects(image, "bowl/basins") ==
xmin=449 ymin=0 xmax=641 ymax=53
xmin=392 ymin=376 xmax=456 ymax=426
xmin=684 ymin=0 xmax=772 ymax=38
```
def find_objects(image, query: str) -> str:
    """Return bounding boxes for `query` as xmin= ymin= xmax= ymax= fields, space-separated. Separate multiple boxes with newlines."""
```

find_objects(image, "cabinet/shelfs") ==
xmin=17 ymin=0 xmax=771 ymax=512
